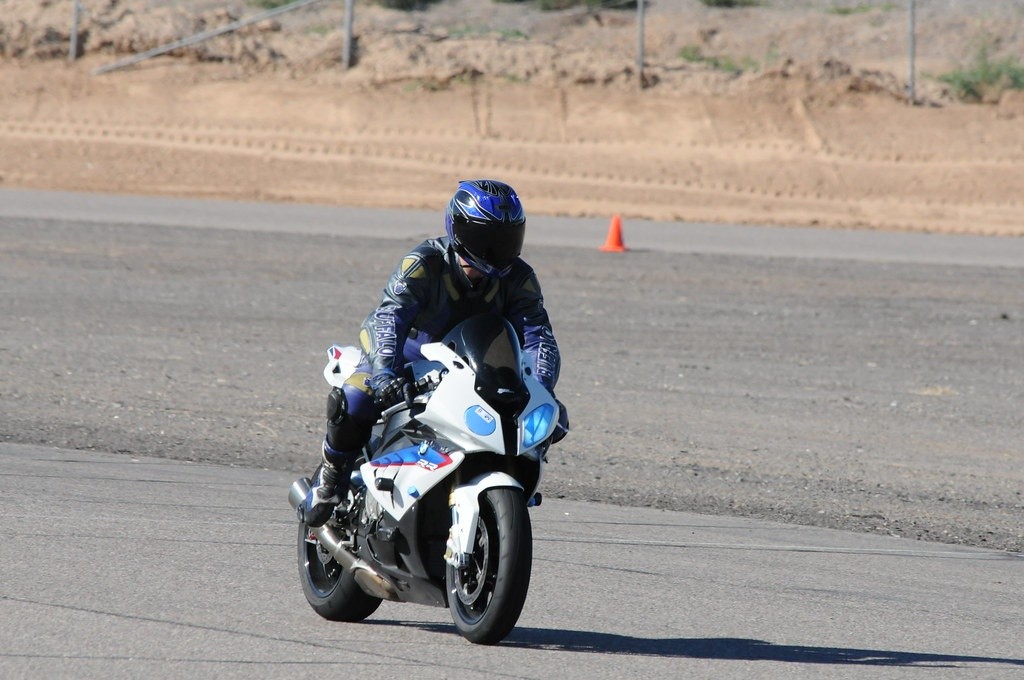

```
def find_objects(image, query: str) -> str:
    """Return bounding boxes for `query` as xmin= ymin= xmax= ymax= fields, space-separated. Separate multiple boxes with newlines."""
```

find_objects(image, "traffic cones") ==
xmin=599 ymin=212 xmax=630 ymax=253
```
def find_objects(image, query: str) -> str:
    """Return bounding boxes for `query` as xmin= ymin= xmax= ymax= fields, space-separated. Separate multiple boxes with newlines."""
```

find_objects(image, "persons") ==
xmin=298 ymin=181 xmax=572 ymax=529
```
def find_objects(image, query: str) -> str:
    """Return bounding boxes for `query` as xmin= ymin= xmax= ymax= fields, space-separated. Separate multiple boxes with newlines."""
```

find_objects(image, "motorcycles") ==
xmin=288 ymin=313 xmax=570 ymax=646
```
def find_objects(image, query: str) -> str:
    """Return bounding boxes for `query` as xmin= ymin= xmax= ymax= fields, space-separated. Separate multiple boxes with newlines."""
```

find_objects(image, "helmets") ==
xmin=445 ymin=180 xmax=526 ymax=279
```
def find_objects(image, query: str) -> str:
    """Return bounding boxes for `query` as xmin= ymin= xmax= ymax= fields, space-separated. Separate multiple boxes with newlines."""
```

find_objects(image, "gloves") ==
xmin=374 ymin=375 xmax=407 ymax=412
xmin=547 ymin=389 xmax=570 ymax=444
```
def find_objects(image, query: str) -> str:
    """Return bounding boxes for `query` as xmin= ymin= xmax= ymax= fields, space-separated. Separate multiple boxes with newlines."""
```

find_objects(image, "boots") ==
xmin=303 ymin=433 xmax=360 ymax=528
